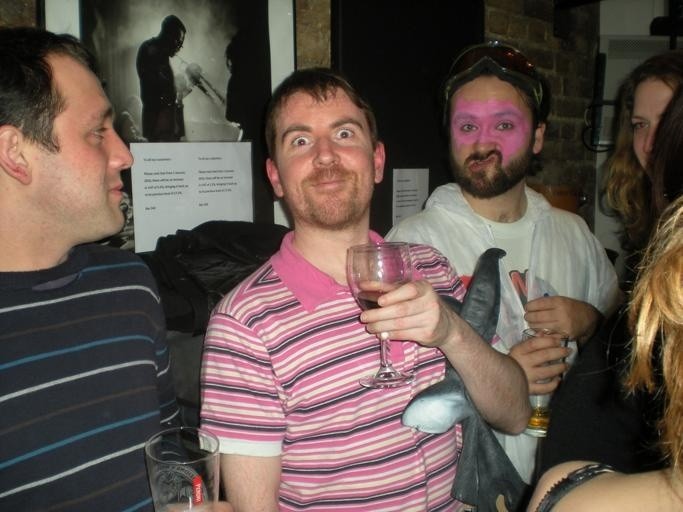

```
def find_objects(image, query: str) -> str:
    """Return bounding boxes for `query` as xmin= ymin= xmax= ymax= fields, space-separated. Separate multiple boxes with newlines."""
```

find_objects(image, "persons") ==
xmin=518 ymin=194 xmax=682 ymax=512
xmin=603 ymin=50 xmax=683 ymax=254
xmin=380 ymin=46 xmax=622 ymax=511
xmin=136 ymin=13 xmax=186 ymax=144
xmin=1 ymin=23 xmax=238 ymax=511
xmin=189 ymin=71 xmax=532 ymax=512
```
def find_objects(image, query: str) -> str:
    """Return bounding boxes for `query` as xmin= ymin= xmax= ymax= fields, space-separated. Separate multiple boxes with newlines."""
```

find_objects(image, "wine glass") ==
xmin=347 ymin=242 xmax=414 ymax=390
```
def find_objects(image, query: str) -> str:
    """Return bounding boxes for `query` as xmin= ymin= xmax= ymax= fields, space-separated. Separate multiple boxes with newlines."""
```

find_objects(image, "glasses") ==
xmin=441 ymin=44 xmax=538 ymax=89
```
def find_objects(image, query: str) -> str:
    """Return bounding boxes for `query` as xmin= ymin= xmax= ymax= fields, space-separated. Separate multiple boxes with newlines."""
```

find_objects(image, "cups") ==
xmin=143 ymin=426 xmax=219 ymax=512
xmin=521 ymin=329 xmax=568 ymax=436
